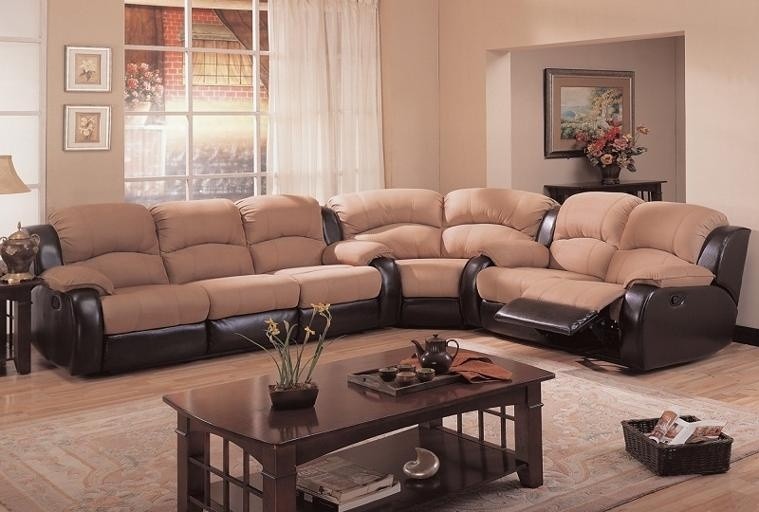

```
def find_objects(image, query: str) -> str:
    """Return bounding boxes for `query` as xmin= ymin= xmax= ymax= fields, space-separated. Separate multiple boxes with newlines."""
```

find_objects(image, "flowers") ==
xmin=124 ymin=63 xmax=165 ymax=110
xmin=576 ymin=117 xmax=651 ymax=173
xmin=233 ymin=302 xmax=333 ymax=388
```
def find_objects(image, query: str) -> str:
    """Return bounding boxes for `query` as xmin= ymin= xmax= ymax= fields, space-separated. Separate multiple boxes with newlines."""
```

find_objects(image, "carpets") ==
xmin=2 ymin=337 xmax=759 ymax=508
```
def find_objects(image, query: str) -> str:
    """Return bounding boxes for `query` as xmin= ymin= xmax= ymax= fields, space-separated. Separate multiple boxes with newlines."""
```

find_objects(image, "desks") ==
xmin=543 ymin=181 xmax=668 ymax=201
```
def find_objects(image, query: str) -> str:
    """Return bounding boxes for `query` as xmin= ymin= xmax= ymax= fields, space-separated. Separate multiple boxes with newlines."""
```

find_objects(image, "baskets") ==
xmin=621 ymin=415 xmax=733 ymax=476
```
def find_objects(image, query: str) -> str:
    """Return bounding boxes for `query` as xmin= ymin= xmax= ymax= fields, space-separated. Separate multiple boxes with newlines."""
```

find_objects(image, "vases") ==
xmin=267 ymin=382 xmax=320 ymax=410
xmin=124 ymin=101 xmax=151 ymax=125
xmin=598 ymin=165 xmax=621 ymax=185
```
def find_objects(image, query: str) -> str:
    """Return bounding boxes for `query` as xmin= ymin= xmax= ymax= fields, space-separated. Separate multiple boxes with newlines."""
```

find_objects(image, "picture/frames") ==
xmin=62 ymin=44 xmax=112 ymax=151
xmin=543 ymin=68 xmax=639 ymax=159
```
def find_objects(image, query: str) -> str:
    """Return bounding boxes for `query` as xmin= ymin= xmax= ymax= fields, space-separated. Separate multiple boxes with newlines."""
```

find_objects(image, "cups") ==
xmin=377 ymin=363 xmax=436 ymax=386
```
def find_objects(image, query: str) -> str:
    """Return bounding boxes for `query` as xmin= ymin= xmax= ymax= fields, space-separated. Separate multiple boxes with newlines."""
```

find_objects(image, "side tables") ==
xmin=0 ymin=277 xmax=42 ymax=377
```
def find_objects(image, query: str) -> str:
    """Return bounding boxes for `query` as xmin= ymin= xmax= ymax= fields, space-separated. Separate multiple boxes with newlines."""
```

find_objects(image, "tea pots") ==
xmin=411 ymin=334 xmax=462 ymax=374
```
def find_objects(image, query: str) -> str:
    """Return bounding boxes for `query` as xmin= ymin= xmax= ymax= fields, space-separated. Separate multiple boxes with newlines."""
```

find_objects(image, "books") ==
xmin=649 ymin=408 xmax=727 ymax=448
xmin=295 ymin=479 xmax=402 ymax=512
xmin=291 ymin=452 xmax=396 ymax=501
